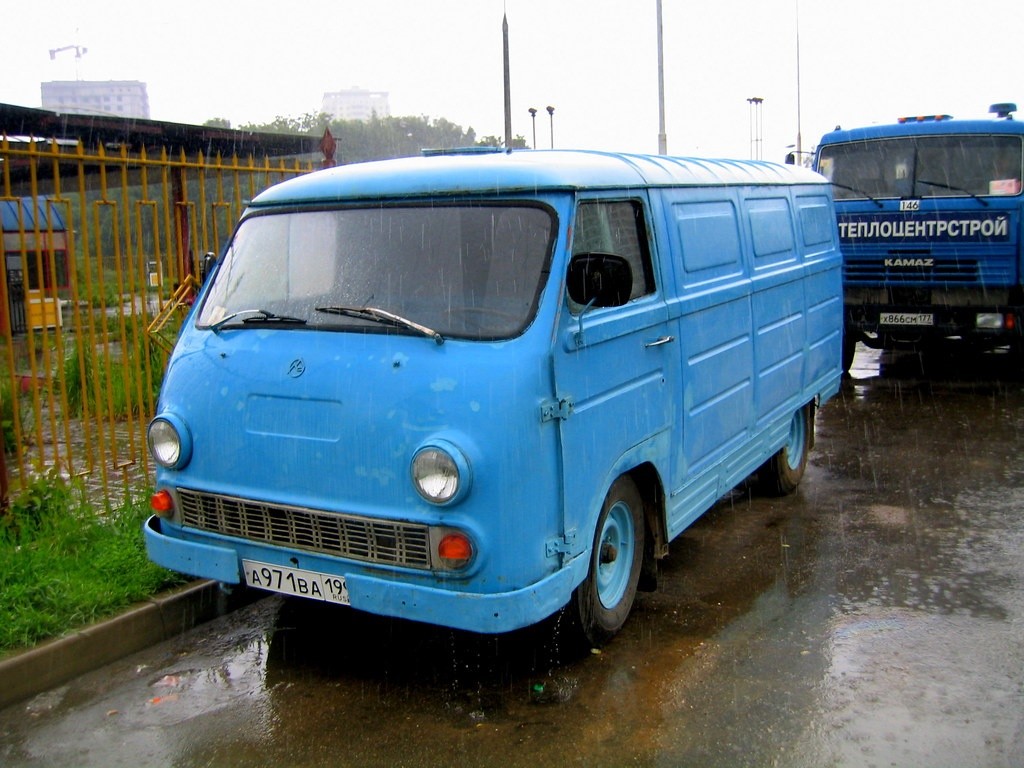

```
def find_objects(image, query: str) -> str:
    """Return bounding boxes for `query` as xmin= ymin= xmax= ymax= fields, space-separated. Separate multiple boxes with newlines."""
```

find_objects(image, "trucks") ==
xmin=785 ymin=114 xmax=1024 ymax=375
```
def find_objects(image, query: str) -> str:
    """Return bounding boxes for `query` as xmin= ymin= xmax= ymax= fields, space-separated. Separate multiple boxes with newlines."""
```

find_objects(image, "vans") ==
xmin=141 ymin=145 xmax=843 ymax=642
